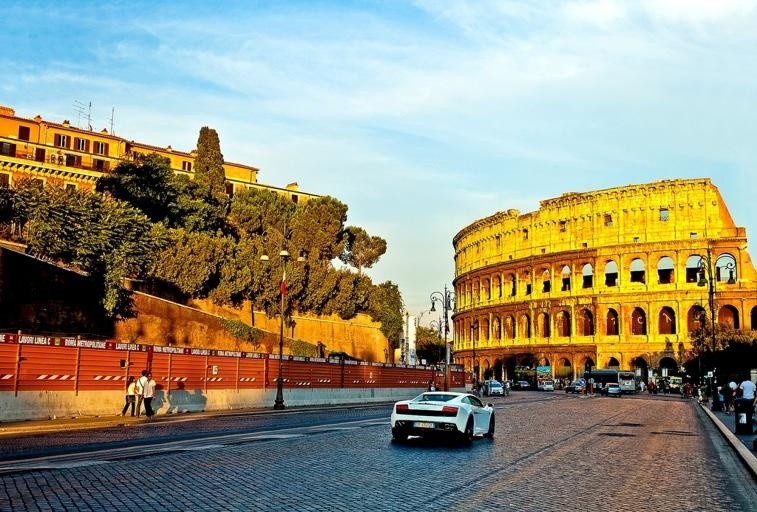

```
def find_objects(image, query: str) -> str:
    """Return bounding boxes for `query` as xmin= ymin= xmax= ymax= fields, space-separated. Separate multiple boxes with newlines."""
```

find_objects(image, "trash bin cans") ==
xmin=733 ymin=398 xmax=755 ymax=435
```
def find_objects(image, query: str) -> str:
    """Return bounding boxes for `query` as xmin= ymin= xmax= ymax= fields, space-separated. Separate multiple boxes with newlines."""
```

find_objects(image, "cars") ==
xmin=478 ymin=377 xmax=622 ymax=399
xmin=663 ymin=375 xmax=683 ymax=392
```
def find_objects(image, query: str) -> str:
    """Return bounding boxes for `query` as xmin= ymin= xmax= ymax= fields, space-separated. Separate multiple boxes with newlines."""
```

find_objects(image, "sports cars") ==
xmin=387 ymin=390 xmax=498 ymax=447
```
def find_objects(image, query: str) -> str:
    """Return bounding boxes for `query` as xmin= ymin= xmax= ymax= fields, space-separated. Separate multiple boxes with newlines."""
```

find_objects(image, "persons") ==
xmin=122 ymin=376 xmax=136 ymax=417
xmin=470 ymin=382 xmax=487 ymax=398
xmin=647 ymin=379 xmax=654 ymax=394
xmin=640 ymin=379 xmax=645 ymax=392
xmin=663 ymin=377 xmax=671 ymax=396
xmin=501 ymin=381 xmax=511 ymax=396
xmin=140 ymin=372 xmax=156 ymax=419
xmin=717 ymin=375 xmax=757 ymax=416
xmin=427 ymin=380 xmax=441 ymax=392
xmin=136 ymin=370 xmax=148 ymax=418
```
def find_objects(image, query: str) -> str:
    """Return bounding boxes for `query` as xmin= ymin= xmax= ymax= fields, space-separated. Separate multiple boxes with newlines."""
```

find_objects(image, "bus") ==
xmin=581 ymin=369 xmax=636 ymax=394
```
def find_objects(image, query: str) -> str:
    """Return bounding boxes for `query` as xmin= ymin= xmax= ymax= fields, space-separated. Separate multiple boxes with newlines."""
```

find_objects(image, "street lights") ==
xmin=427 ymin=283 xmax=460 ymax=392
xmin=255 ymin=247 xmax=310 ymax=411
xmin=694 ymin=250 xmax=738 ymax=414
